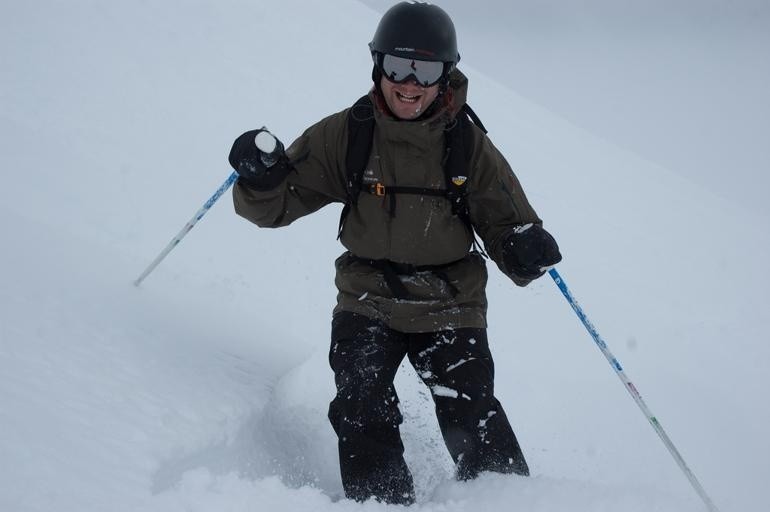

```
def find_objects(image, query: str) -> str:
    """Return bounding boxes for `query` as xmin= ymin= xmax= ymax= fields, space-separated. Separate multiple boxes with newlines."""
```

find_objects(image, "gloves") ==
xmin=503 ymin=223 xmax=562 ymax=281
xmin=229 ymin=129 xmax=289 ymax=183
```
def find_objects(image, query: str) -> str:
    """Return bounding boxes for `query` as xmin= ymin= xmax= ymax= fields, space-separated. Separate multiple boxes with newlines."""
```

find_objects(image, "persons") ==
xmin=227 ymin=0 xmax=565 ymax=507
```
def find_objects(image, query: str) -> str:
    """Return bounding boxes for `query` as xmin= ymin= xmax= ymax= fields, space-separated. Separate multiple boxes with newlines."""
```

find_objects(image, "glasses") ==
xmin=376 ymin=51 xmax=452 ymax=88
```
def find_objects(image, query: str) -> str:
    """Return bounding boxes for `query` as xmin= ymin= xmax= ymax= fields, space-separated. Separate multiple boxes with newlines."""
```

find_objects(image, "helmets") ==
xmin=368 ymin=1 xmax=460 ymax=65
